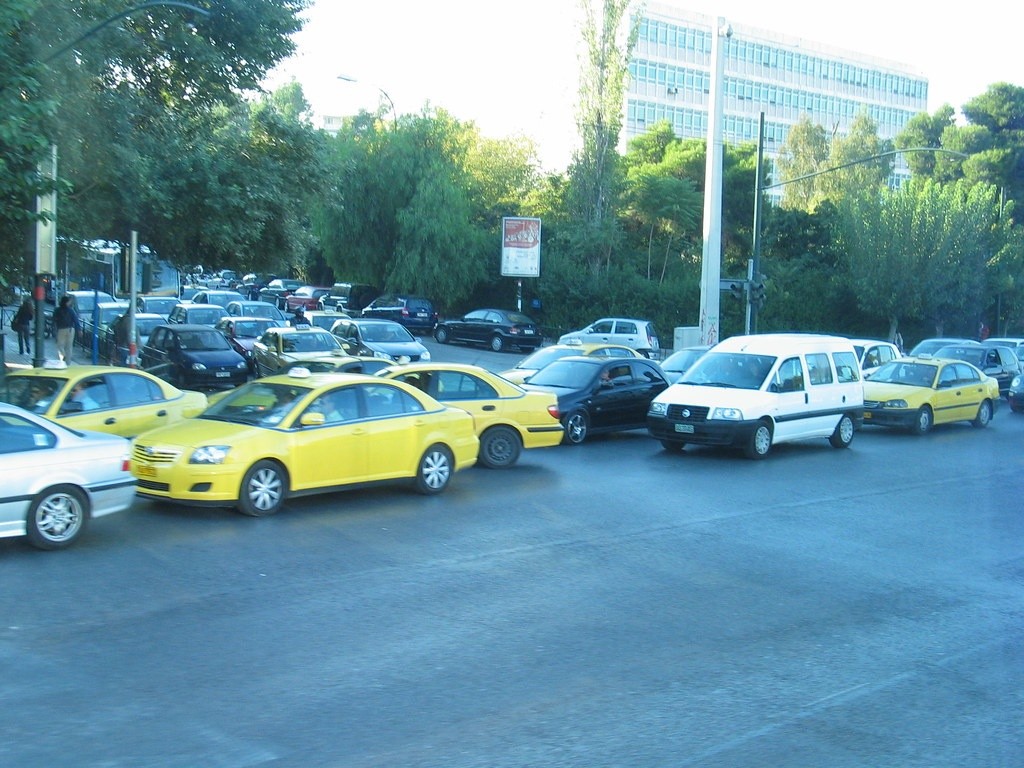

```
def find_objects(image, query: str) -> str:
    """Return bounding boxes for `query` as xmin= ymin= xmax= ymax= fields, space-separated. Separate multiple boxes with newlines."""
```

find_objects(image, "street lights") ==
xmin=338 ymin=75 xmax=398 ymax=133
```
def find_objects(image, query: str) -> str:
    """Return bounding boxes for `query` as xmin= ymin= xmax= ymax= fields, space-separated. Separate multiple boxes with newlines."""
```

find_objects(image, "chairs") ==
xmin=418 ymin=372 xmax=430 ymax=394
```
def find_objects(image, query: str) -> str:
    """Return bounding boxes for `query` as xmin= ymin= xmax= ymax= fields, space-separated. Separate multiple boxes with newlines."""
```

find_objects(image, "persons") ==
xmin=28 ymin=378 xmax=51 ymax=407
xmin=70 ymin=382 xmax=101 ymax=412
xmin=14 ymin=299 xmax=34 ymax=355
xmin=600 ymin=370 xmax=613 ymax=386
xmin=52 ymin=296 xmax=82 ymax=365
xmin=289 ymin=305 xmax=312 ymax=327
xmin=319 ymin=391 xmax=344 ymax=424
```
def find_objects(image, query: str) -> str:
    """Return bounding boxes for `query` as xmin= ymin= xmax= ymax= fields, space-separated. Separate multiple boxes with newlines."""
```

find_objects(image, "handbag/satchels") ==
xmin=11 ymin=317 xmax=19 ymax=332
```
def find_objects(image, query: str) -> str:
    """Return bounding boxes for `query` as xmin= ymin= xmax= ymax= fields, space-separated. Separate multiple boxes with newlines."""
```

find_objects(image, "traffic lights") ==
xmin=141 ymin=261 xmax=163 ymax=294
xmin=730 ymin=283 xmax=744 ymax=303
xmin=749 ymin=280 xmax=764 ymax=305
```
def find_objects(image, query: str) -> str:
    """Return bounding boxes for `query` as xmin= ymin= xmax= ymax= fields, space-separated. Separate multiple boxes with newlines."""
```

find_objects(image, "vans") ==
xmin=646 ymin=333 xmax=865 ymax=460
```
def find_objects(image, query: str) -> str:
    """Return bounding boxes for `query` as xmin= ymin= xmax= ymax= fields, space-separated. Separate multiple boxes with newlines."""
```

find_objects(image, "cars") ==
xmin=496 ymin=339 xmax=661 ymax=386
xmin=361 ymin=295 xmax=439 ymax=334
xmin=0 ymin=365 xmax=207 ymax=446
xmin=284 ymin=286 xmax=332 ymax=311
xmin=208 ymin=300 xmax=444 ymax=415
xmin=519 ymin=356 xmax=672 ymax=444
xmin=180 ymin=264 xmax=306 ymax=310
xmin=316 ymin=283 xmax=382 ymax=318
xmin=131 ymin=365 xmax=480 ymax=516
xmin=660 ymin=345 xmax=715 ymax=384
xmin=850 ymin=337 xmax=1024 ymax=436
xmin=363 ymin=362 xmax=564 ymax=469
xmin=0 ymin=401 xmax=139 ymax=550
xmin=66 ymin=291 xmax=249 ymax=390
xmin=433 ymin=308 xmax=662 ymax=360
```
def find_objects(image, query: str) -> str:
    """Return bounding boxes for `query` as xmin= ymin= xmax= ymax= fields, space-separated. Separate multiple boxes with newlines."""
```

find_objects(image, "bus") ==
xmin=23 ymin=236 xmax=181 ymax=303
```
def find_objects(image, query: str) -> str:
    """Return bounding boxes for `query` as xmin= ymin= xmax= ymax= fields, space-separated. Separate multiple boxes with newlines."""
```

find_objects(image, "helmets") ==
xmin=295 ymin=307 xmax=305 ymax=315
